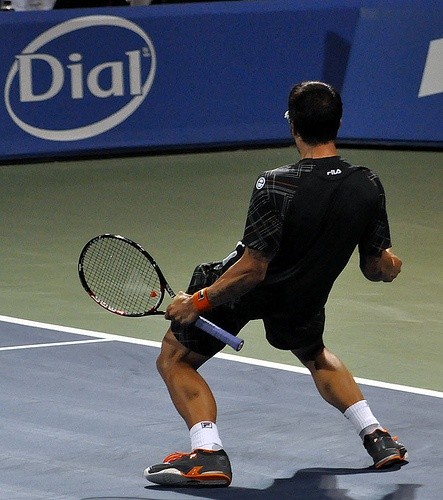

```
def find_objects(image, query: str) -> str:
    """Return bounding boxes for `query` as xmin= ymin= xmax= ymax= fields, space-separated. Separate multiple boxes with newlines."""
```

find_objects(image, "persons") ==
xmin=143 ymin=81 xmax=408 ymax=487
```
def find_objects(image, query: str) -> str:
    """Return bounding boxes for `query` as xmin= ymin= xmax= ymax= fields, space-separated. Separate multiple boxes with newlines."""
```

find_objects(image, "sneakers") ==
xmin=144 ymin=448 xmax=232 ymax=488
xmin=363 ymin=429 xmax=409 ymax=470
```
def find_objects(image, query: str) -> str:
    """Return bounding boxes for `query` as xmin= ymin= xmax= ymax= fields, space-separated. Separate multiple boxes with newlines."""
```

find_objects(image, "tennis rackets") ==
xmin=78 ymin=233 xmax=246 ymax=351
